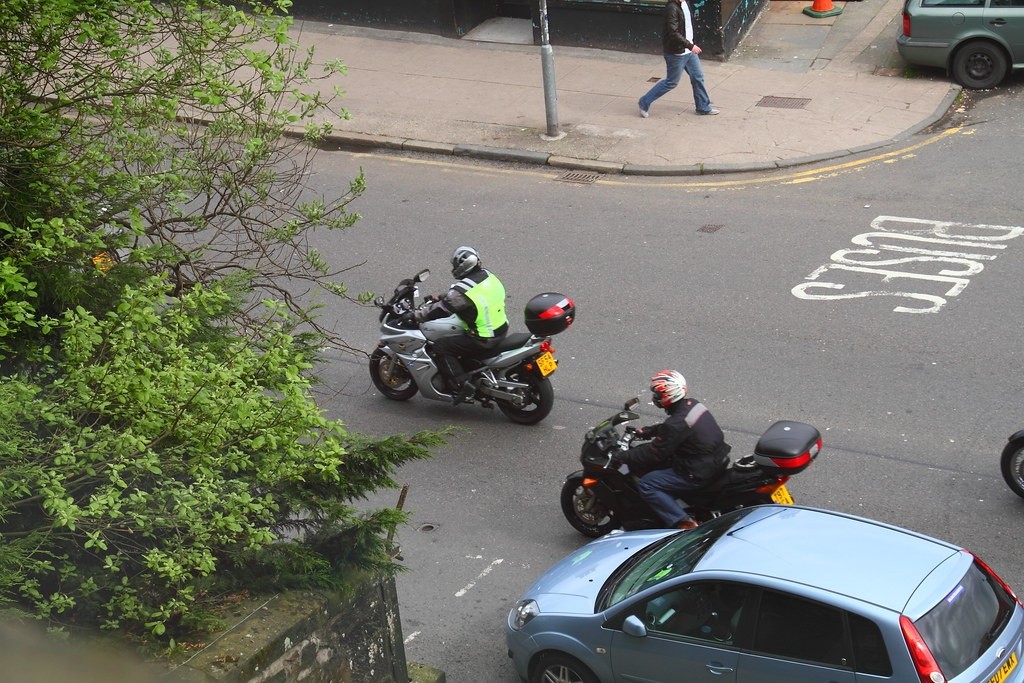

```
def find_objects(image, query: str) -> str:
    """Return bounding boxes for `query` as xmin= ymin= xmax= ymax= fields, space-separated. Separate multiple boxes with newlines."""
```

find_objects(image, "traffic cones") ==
xmin=802 ymin=0 xmax=842 ymax=18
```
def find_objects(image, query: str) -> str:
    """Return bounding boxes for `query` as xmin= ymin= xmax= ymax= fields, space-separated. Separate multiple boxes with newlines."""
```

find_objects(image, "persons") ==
xmin=638 ymin=0 xmax=720 ymax=119
xmin=612 ymin=369 xmax=731 ymax=530
xmin=400 ymin=245 xmax=508 ymax=406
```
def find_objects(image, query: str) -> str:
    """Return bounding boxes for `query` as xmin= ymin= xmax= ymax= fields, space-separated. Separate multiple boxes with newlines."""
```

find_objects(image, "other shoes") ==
xmin=676 ymin=517 xmax=699 ymax=529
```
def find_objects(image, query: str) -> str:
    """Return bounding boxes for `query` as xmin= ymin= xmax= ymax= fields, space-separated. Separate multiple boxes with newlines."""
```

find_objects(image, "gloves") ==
xmin=611 ymin=448 xmax=625 ymax=466
xmin=637 ymin=427 xmax=656 ymax=440
xmin=401 ymin=312 xmax=416 ymax=326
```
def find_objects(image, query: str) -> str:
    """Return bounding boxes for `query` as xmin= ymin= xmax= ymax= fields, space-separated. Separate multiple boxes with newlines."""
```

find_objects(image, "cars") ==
xmin=506 ymin=504 xmax=1024 ymax=683
xmin=897 ymin=0 xmax=1024 ymax=89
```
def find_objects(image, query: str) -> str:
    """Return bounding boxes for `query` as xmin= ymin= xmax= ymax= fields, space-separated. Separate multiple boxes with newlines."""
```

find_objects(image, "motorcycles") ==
xmin=999 ymin=430 xmax=1024 ymax=501
xmin=561 ymin=397 xmax=823 ymax=537
xmin=369 ymin=269 xmax=576 ymax=424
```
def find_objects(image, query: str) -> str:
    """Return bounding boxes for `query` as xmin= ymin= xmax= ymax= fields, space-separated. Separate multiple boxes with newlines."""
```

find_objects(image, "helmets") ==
xmin=650 ymin=370 xmax=688 ymax=408
xmin=451 ymin=245 xmax=482 ymax=280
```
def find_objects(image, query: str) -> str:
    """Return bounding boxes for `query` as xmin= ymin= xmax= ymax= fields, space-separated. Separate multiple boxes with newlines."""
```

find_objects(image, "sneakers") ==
xmin=453 ymin=380 xmax=476 ymax=405
xmin=639 ymin=100 xmax=649 ymax=119
xmin=697 ymin=108 xmax=721 ymax=115
